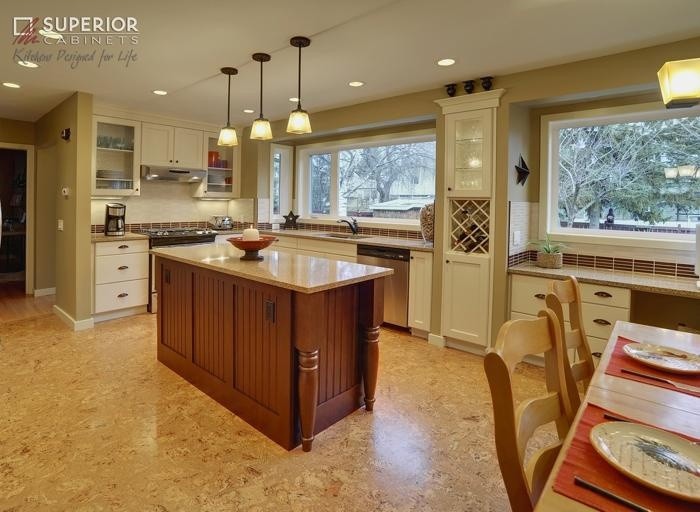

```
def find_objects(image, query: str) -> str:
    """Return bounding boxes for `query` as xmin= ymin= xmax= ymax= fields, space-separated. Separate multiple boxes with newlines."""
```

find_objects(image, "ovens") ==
xmin=356 ymin=254 xmax=409 ymax=329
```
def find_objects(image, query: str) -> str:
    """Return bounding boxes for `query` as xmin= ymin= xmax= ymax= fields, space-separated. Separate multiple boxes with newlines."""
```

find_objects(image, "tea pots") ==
xmin=208 ymin=215 xmax=234 ymax=230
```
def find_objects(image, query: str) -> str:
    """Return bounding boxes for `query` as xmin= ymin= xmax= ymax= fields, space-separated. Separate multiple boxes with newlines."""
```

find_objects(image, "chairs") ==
xmin=483 ymin=274 xmax=595 ymax=511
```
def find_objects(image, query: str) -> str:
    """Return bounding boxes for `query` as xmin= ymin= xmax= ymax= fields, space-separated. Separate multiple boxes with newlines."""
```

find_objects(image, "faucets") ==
xmin=336 ymin=216 xmax=358 ymax=235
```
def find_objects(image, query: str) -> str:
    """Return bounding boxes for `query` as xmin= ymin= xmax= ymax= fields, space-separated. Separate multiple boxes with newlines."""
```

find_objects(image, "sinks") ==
xmin=318 ymin=233 xmax=373 ymax=239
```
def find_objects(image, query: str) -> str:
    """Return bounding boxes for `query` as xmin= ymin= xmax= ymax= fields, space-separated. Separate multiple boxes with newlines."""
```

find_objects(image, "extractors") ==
xmin=139 ymin=164 xmax=209 ymax=184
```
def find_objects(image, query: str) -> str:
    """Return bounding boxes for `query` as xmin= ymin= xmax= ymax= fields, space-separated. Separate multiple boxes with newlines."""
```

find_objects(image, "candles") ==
xmin=242 ymin=229 xmax=260 ymax=241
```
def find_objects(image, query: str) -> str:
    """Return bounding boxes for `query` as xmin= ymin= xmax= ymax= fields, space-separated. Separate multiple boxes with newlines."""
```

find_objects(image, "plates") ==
xmin=620 ymin=340 xmax=700 ymax=374
xmin=96 ymin=168 xmax=126 ymax=178
xmin=208 ymin=172 xmax=226 ymax=193
xmin=590 ymin=419 xmax=700 ymax=505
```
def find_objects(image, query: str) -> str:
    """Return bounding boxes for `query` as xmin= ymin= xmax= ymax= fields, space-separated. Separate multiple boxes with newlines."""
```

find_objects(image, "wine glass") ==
xmin=226 ymin=235 xmax=280 ymax=261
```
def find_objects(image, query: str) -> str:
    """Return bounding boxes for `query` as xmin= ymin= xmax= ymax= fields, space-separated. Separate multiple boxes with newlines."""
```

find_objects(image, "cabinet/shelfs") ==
xmin=141 ymin=123 xmax=204 ymax=170
xmin=575 ymin=283 xmax=632 ymax=371
xmin=407 ymin=250 xmax=432 ymax=332
xmin=191 ymin=132 xmax=240 ymax=201
xmin=91 ymin=114 xmax=141 ymax=198
xmin=264 ymin=237 xmax=300 ymax=256
xmin=442 ymin=108 xmax=492 ymax=343
xmin=297 ymin=239 xmax=357 ymax=263
xmin=92 ymin=240 xmax=150 ymax=313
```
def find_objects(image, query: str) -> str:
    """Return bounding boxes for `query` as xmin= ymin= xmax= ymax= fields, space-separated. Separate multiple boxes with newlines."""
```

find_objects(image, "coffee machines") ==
xmin=104 ymin=202 xmax=125 ymax=235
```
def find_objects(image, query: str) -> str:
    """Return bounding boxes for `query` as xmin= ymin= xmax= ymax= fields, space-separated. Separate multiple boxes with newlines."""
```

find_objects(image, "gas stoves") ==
xmin=135 ymin=227 xmax=216 ymax=238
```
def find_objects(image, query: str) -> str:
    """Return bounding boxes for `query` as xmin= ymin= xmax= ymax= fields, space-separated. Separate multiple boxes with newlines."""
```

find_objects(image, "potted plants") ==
xmin=531 ymin=232 xmax=573 ymax=270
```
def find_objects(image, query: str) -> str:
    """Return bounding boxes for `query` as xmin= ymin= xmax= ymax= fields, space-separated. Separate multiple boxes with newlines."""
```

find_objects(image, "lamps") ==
xmin=216 ymin=36 xmax=313 ymax=147
xmin=656 ymin=57 xmax=700 ymax=110
